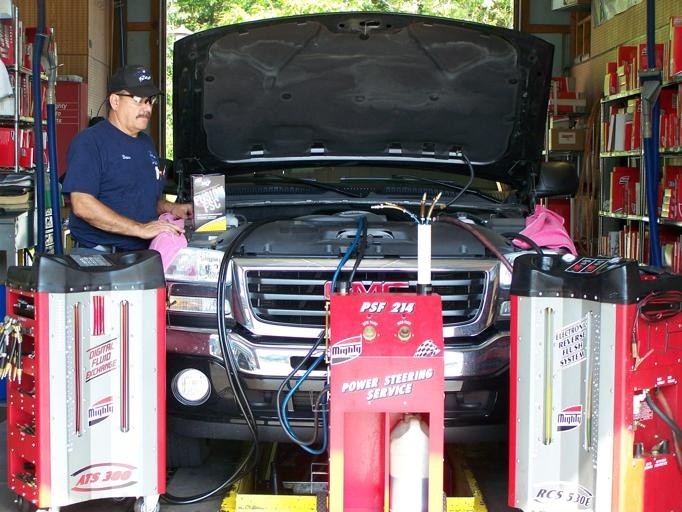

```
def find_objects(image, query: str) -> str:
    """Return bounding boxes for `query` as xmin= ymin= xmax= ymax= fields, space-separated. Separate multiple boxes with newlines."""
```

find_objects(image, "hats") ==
xmin=107 ymin=62 xmax=167 ymax=101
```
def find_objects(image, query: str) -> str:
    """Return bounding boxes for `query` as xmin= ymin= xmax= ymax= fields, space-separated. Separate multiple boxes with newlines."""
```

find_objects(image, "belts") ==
xmin=70 ymin=237 xmax=144 ymax=258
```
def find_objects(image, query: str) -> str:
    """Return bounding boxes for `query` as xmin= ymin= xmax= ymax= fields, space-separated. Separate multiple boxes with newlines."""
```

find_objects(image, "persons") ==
xmin=61 ymin=64 xmax=193 ymax=257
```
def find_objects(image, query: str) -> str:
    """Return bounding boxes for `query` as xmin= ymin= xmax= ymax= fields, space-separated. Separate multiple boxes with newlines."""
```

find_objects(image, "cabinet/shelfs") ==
xmin=539 ymin=72 xmax=681 ymax=274
xmin=1 ymin=3 xmax=59 ymax=175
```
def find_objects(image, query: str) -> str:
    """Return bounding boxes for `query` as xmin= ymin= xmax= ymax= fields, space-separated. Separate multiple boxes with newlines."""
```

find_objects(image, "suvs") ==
xmin=148 ymin=10 xmax=561 ymax=478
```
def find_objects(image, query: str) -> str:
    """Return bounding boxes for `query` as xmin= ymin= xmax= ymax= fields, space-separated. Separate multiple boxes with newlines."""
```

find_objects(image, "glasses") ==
xmin=111 ymin=91 xmax=158 ymax=105
